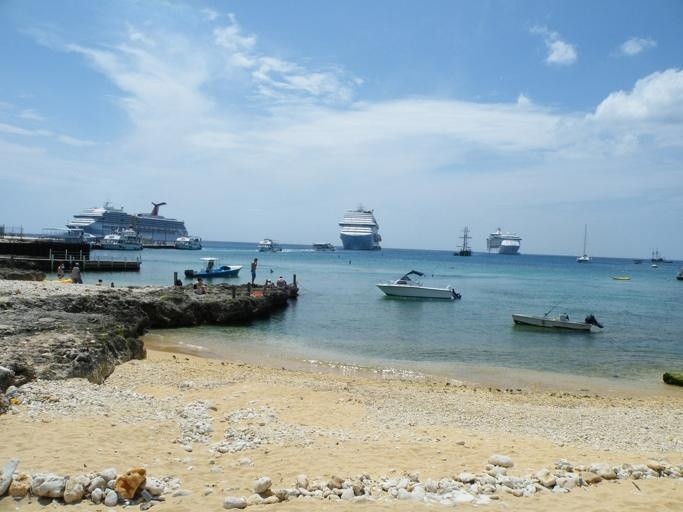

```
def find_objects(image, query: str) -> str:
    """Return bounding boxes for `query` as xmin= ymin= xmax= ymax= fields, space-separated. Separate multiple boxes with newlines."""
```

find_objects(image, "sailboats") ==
xmin=458 ymin=226 xmax=472 ymax=257
xmin=576 ymin=225 xmax=590 ymax=263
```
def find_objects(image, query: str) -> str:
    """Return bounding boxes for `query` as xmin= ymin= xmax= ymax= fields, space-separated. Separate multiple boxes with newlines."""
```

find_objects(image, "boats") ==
xmin=173 ymin=236 xmax=202 ymax=250
xmin=314 ymin=243 xmax=336 ymax=252
xmin=256 ymin=240 xmax=281 ymax=252
xmin=376 ymin=271 xmax=462 ymax=299
xmin=186 ymin=266 xmax=243 ymax=279
xmin=93 ymin=228 xmax=145 ymax=251
xmin=487 ymin=230 xmax=521 ymax=254
xmin=512 ymin=313 xmax=603 ymax=331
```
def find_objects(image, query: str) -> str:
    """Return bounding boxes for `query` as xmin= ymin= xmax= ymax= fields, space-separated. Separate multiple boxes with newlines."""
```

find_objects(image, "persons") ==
xmin=250 ymin=258 xmax=257 ymax=284
xmin=57 ymin=264 xmax=63 ymax=280
xmin=266 ymin=279 xmax=275 ymax=288
xmin=70 ymin=262 xmax=82 ymax=284
xmin=95 ymin=279 xmax=102 ymax=286
xmin=276 ymin=276 xmax=286 ymax=288
xmin=193 ymin=277 xmax=207 ymax=294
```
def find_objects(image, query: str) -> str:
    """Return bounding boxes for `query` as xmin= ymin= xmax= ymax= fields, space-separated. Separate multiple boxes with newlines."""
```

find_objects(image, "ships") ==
xmin=66 ymin=202 xmax=188 ymax=249
xmin=338 ymin=210 xmax=382 ymax=250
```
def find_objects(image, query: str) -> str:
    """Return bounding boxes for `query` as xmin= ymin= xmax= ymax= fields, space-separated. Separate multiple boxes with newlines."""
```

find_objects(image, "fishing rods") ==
xmin=541 ymin=290 xmax=577 ymax=318
